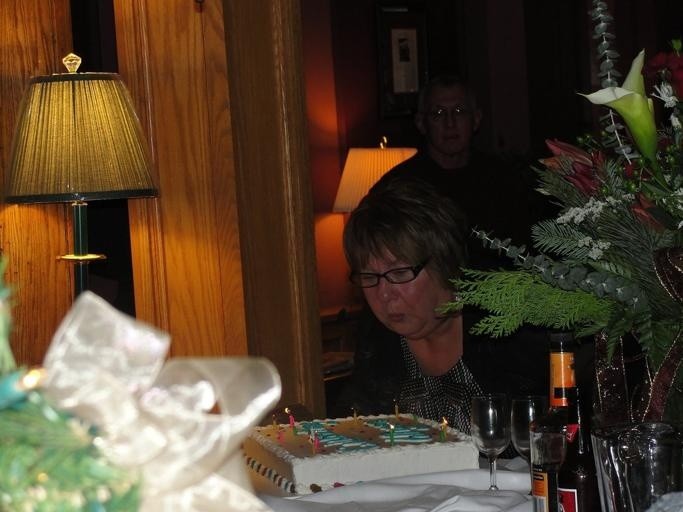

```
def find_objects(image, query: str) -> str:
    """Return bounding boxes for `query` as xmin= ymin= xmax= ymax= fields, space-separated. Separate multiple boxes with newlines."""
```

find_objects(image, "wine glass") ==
xmin=470 ymin=393 xmax=541 ymax=496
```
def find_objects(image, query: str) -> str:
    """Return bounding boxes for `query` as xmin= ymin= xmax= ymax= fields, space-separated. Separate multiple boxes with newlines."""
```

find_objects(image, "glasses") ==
xmin=347 ymin=259 xmax=431 ymax=288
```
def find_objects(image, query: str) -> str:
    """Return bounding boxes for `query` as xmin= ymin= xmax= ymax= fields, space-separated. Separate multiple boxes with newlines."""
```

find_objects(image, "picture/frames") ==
xmin=375 ymin=6 xmax=430 ymax=120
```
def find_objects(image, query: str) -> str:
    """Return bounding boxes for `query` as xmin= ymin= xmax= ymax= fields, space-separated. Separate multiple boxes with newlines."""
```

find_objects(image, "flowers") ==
xmin=431 ymin=0 xmax=683 ymax=382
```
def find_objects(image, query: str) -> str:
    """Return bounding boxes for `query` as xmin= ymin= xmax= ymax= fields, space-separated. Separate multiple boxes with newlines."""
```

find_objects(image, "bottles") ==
xmin=529 ymin=332 xmax=597 ymax=512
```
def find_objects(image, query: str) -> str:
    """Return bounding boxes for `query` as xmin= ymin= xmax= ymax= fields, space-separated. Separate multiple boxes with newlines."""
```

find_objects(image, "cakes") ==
xmin=242 ymin=412 xmax=481 ymax=497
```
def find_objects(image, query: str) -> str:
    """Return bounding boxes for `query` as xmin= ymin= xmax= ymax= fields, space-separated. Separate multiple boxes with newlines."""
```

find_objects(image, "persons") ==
xmin=342 ymin=177 xmax=597 ymax=460
xmin=368 ymin=72 xmax=514 ymax=233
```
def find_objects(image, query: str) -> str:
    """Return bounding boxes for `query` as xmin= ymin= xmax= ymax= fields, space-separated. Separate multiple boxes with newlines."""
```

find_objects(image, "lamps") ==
xmin=329 ymin=133 xmax=416 ymax=216
xmin=6 ymin=52 xmax=161 ymax=296
